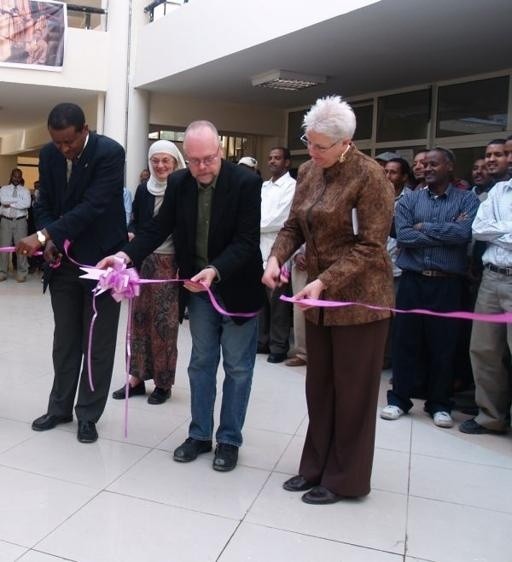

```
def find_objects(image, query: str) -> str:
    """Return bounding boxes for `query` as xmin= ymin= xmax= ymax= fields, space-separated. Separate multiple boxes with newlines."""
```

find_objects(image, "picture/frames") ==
xmin=0 ymin=0 xmax=66 ymax=71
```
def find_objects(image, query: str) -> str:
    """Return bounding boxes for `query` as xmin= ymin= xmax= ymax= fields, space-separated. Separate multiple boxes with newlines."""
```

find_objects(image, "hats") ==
xmin=373 ymin=152 xmax=401 ymax=161
xmin=238 ymin=157 xmax=258 ymax=172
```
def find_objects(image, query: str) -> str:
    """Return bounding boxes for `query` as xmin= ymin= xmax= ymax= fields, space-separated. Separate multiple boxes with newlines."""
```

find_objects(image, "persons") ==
xmin=112 ymin=139 xmax=187 ymax=405
xmin=15 ymin=102 xmax=127 ymax=443
xmin=122 ymin=137 xmax=512 ymax=434
xmin=261 ymin=94 xmax=397 ymax=504
xmin=0 ymin=168 xmax=41 ymax=283
xmin=94 ymin=120 xmax=267 ymax=472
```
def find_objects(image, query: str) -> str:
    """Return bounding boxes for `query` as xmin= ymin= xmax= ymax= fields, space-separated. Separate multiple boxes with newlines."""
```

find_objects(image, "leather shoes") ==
xmin=32 ymin=412 xmax=74 ymax=431
xmin=258 ymin=346 xmax=305 ymax=366
xmin=172 ymin=435 xmax=213 ymax=462
xmin=147 ymin=386 xmax=172 ymax=405
xmin=459 ymin=418 xmax=495 ymax=434
xmin=112 ymin=380 xmax=144 ymax=398
xmin=302 ymin=481 xmax=344 ymax=504
xmin=283 ymin=475 xmax=317 ymax=492
xmin=214 ymin=443 xmax=238 ymax=472
xmin=77 ymin=420 xmax=98 ymax=442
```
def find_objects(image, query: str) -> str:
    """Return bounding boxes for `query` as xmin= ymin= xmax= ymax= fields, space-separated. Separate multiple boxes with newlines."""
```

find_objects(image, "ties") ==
xmin=67 ymin=158 xmax=78 ymax=181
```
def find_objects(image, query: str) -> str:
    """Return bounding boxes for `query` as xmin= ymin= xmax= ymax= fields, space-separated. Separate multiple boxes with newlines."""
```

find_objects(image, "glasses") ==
xmin=300 ymin=133 xmax=340 ymax=152
xmin=188 ymin=145 xmax=220 ymax=167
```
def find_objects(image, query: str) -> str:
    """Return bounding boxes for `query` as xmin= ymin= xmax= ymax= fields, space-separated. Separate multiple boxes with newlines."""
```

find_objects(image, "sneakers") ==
xmin=381 ymin=403 xmax=404 ymax=420
xmin=433 ymin=412 xmax=454 ymax=428
xmin=16 ymin=275 xmax=27 ymax=281
xmin=0 ymin=277 xmax=8 ymax=281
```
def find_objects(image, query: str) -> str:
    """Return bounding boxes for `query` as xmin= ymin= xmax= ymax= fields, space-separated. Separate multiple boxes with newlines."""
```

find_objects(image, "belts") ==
xmin=417 ymin=270 xmax=453 ymax=278
xmin=3 ymin=215 xmax=27 ymax=220
xmin=486 ymin=262 xmax=512 ymax=276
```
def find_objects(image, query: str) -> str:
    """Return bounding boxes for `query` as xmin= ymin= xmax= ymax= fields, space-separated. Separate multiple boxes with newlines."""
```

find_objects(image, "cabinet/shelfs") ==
xmin=283 ymin=70 xmax=512 ymax=167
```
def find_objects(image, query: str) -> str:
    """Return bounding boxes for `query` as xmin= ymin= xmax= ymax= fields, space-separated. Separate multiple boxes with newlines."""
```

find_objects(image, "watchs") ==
xmin=36 ymin=231 xmax=45 ymax=247
